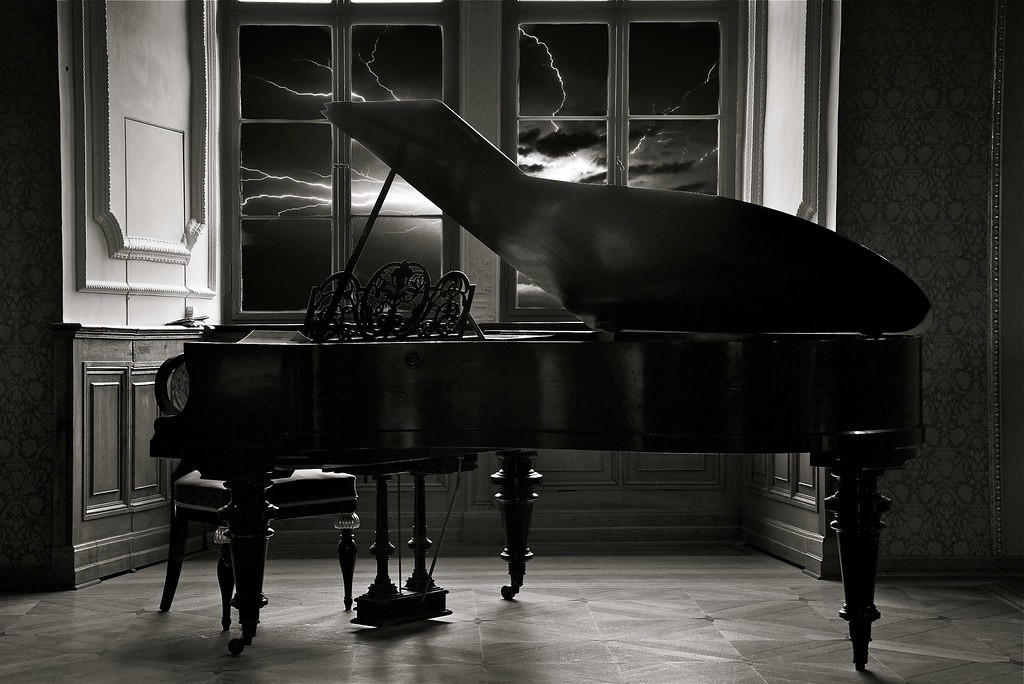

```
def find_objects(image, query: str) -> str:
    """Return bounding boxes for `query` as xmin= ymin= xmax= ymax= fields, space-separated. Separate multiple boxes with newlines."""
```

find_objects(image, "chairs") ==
xmin=150 ymin=353 xmax=358 ymax=631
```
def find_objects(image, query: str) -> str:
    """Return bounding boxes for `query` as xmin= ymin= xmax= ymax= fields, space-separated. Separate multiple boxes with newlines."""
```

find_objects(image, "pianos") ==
xmin=148 ymin=96 xmax=936 ymax=673
